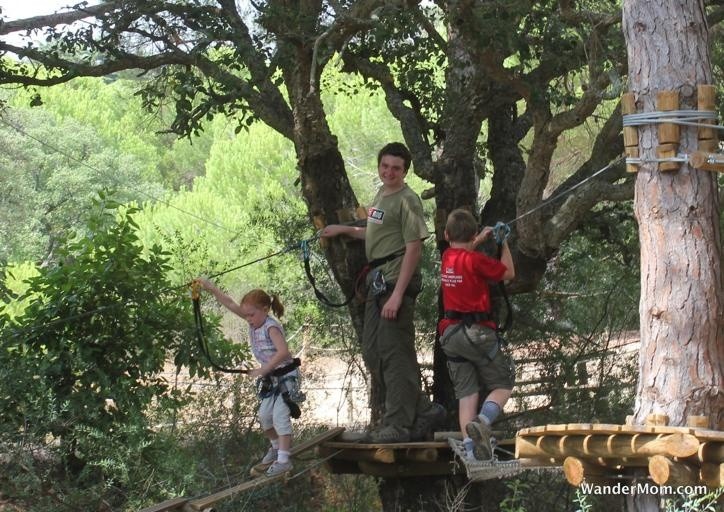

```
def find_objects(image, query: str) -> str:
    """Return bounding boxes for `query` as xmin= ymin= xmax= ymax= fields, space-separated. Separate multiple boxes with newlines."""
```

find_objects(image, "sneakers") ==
xmin=462 ymin=415 xmax=497 ymax=461
xmin=262 ymin=448 xmax=293 ymax=477
xmin=367 ymin=402 xmax=447 ymax=443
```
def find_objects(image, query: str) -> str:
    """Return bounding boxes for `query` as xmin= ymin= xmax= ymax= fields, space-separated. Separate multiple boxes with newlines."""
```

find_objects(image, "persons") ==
xmin=195 ymin=278 xmax=297 ymax=476
xmin=438 ymin=209 xmax=515 ymax=461
xmin=321 ymin=143 xmax=444 ymax=442
